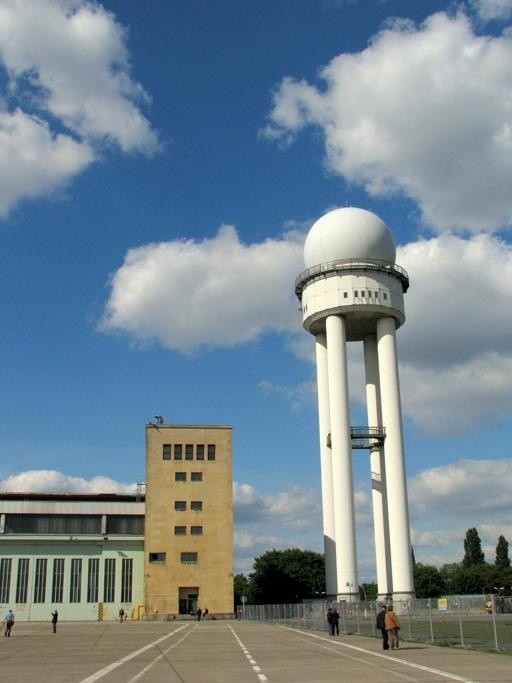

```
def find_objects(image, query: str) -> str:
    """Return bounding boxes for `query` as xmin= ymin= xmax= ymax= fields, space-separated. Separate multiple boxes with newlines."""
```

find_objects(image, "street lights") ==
xmin=314 ymin=591 xmax=328 ymax=632
xmin=346 ymin=582 xmax=353 ymax=621
xmin=493 ymin=585 xmax=504 ymax=596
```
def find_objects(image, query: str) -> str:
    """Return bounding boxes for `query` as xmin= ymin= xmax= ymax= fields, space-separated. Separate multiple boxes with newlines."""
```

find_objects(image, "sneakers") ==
xmin=384 ymin=645 xmax=400 ymax=650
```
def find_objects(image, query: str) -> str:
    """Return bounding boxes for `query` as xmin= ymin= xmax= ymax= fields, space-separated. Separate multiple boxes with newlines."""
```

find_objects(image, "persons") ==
xmin=326 ymin=607 xmax=334 ymax=635
xmin=376 ymin=604 xmax=389 ymax=649
xmin=203 ymin=606 xmax=208 ymax=616
xmin=118 ymin=607 xmax=124 ymax=623
xmin=2 ymin=609 xmax=14 ymax=637
xmin=384 ymin=604 xmax=402 ymax=649
xmin=236 ymin=605 xmax=242 ymax=621
xmin=332 ymin=607 xmax=340 ymax=635
xmin=195 ymin=606 xmax=202 ymax=620
xmin=50 ymin=608 xmax=59 ymax=632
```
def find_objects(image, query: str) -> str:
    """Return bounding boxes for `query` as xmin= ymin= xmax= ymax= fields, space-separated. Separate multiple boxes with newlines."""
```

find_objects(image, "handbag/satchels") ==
xmin=394 ymin=624 xmax=400 ymax=629
xmin=9 ymin=620 xmax=14 ymax=626
